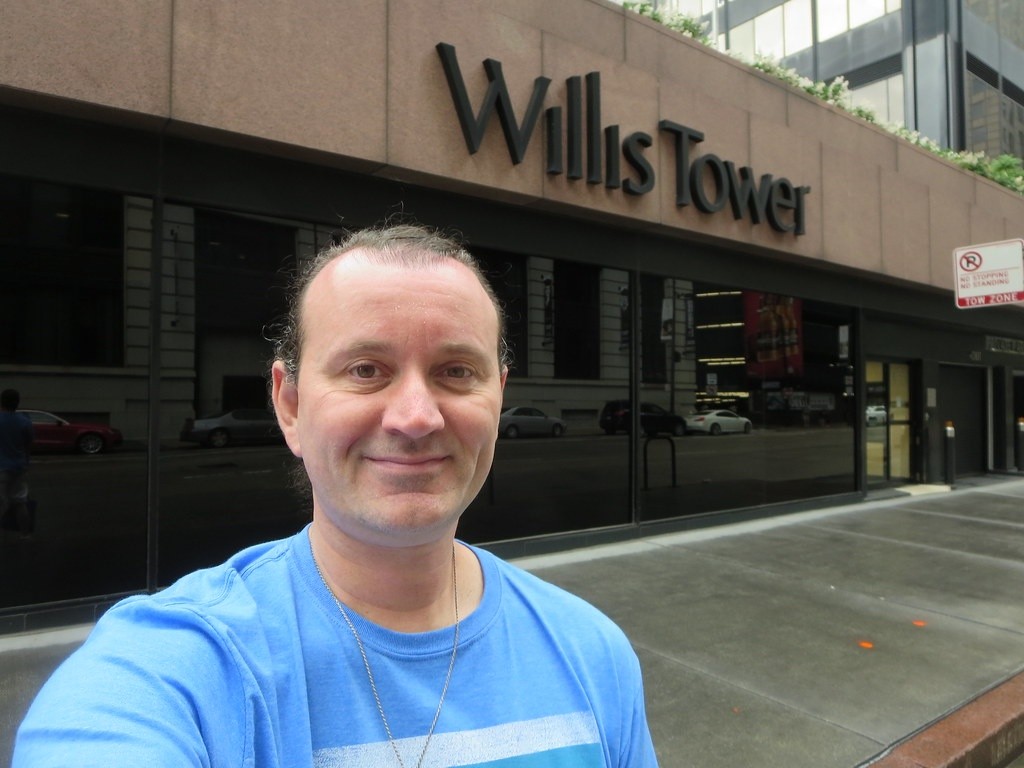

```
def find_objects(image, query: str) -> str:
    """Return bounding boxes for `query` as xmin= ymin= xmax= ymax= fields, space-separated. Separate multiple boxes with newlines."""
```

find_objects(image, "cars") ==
xmin=598 ymin=398 xmax=690 ymax=438
xmin=17 ymin=408 xmax=124 ymax=455
xmin=867 ymin=405 xmax=894 ymax=426
xmin=180 ymin=400 xmax=287 ymax=448
xmin=682 ymin=409 xmax=754 ymax=435
xmin=498 ymin=406 xmax=568 ymax=438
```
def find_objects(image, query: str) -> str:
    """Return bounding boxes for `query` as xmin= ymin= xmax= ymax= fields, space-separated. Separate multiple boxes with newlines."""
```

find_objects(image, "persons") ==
xmin=0 ymin=389 xmax=33 ymax=542
xmin=13 ymin=226 xmax=660 ymax=768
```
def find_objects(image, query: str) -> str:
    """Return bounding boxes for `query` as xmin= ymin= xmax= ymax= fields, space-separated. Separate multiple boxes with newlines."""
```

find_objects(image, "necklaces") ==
xmin=311 ymin=541 xmax=459 ymax=768
